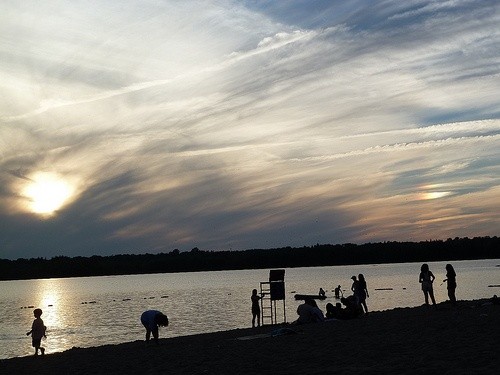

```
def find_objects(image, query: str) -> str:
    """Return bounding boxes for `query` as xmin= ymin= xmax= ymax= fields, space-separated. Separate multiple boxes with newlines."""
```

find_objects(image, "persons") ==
xmin=419 ymin=264 xmax=436 ymax=305
xmin=251 ymin=289 xmax=264 ymax=328
xmin=293 ymin=274 xmax=369 ymax=326
xmin=26 ymin=309 xmax=47 ymax=356
xmin=141 ymin=310 xmax=169 ymax=344
xmin=443 ymin=264 xmax=456 ymax=303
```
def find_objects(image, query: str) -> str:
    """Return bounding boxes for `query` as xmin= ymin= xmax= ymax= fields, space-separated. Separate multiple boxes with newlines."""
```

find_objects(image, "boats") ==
xmin=294 ymin=292 xmax=347 ymax=300
